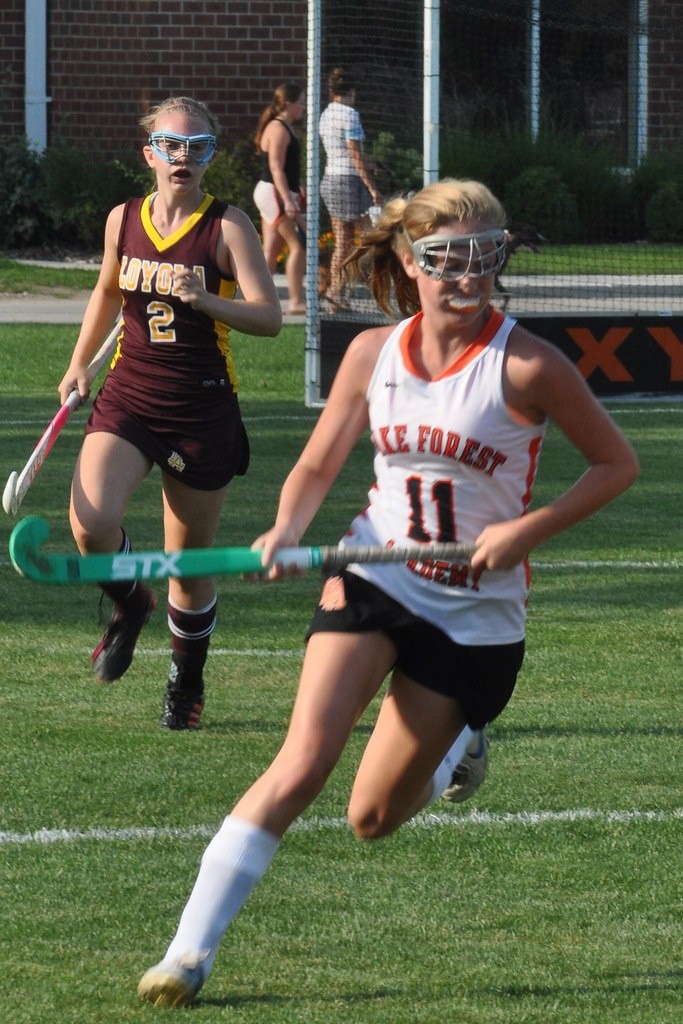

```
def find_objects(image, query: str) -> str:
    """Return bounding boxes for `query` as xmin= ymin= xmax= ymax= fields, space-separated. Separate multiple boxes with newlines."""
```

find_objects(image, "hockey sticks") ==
xmin=8 ymin=513 xmax=480 ymax=586
xmin=2 ymin=316 xmax=125 ymax=517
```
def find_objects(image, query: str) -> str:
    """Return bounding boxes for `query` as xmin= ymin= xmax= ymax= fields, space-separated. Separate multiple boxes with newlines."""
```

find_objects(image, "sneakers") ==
xmin=137 ymin=949 xmax=214 ymax=1009
xmin=441 ymin=729 xmax=487 ymax=802
xmin=92 ymin=580 xmax=156 ymax=684
xmin=160 ymin=654 xmax=208 ymax=731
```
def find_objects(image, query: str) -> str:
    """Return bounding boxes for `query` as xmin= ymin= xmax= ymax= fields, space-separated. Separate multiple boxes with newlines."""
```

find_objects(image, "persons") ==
xmin=248 ymin=80 xmax=316 ymax=315
xmin=57 ymin=97 xmax=281 ymax=731
xmin=138 ymin=183 xmax=641 ymax=1013
xmin=317 ymin=69 xmax=387 ymax=316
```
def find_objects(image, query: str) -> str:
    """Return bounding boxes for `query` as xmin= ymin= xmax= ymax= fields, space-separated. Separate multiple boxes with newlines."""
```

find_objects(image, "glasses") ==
xmin=149 ymin=132 xmax=217 ymax=165
xmin=401 ymin=223 xmax=508 ymax=280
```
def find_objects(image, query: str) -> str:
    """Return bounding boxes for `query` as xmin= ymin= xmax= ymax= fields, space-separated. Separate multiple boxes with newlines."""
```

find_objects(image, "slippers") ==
xmin=290 ymin=301 xmax=327 ymax=316
xmin=322 ymin=290 xmax=353 ymax=313
